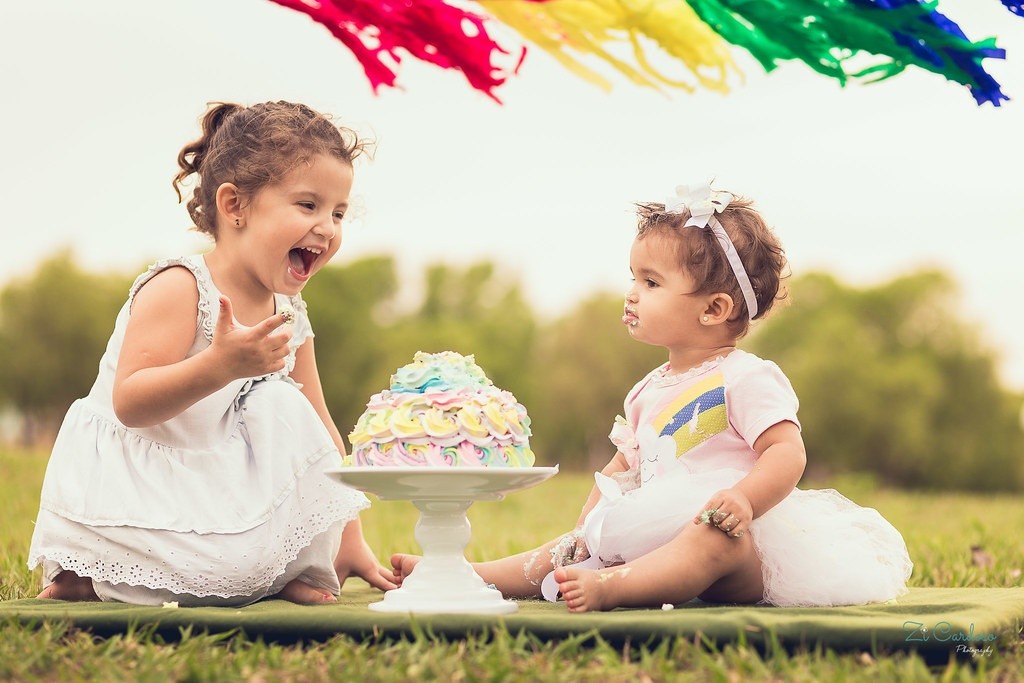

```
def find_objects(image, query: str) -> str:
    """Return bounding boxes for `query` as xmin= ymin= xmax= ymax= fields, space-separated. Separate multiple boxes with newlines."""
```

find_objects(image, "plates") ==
xmin=323 ymin=468 xmax=562 ymax=502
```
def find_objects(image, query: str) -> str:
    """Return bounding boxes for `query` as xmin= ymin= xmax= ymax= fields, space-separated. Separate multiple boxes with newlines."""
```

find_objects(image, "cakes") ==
xmin=342 ymin=350 xmax=536 ymax=468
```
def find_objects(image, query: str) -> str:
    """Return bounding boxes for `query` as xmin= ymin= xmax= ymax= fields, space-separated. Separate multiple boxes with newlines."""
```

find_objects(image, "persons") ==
xmin=20 ymin=98 xmax=399 ymax=610
xmin=390 ymin=173 xmax=913 ymax=610
